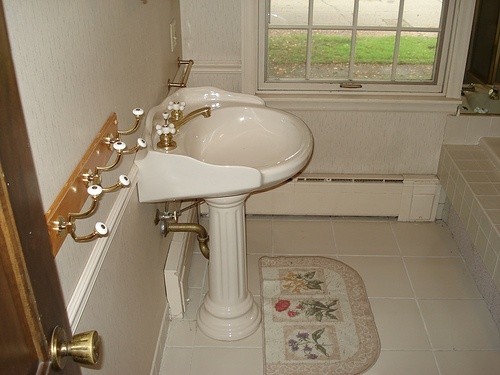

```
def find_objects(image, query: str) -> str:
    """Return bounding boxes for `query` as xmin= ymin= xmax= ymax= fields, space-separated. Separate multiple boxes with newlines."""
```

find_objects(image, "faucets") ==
xmin=164 ymin=106 xmax=212 ymax=135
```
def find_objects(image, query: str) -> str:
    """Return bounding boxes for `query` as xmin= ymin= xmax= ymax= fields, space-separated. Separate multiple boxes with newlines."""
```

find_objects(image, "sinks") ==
xmin=132 ymin=84 xmax=317 ymax=206
